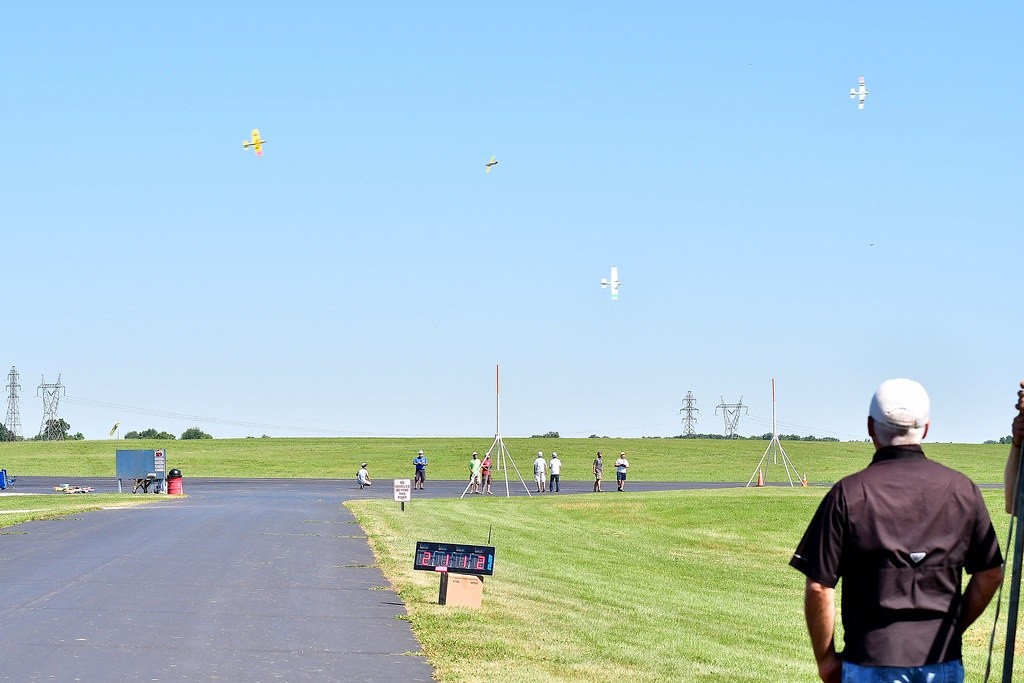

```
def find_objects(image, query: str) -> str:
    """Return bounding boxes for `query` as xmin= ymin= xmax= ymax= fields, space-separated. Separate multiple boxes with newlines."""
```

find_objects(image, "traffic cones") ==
xmin=756 ymin=467 xmax=765 ymax=487
xmin=802 ymin=473 xmax=807 ymax=486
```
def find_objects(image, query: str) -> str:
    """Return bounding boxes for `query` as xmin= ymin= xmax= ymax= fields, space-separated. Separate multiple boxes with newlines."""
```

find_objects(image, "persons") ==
xmin=356 ymin=463 xmax=372 ymax=489
xmin=533 ymin=451 xmax=549 ymax=492
xmin=412 ymin=449 xmax=429 ymax=490
xmin=468 ymin=452 xmax=483 ymax=494
xmin=593 ymin=451 xmax=604 ymax=492
xmin=614 ymin=452 xmax=629 ymax=492
xmin=548 ymin=452 xmax=562 ymax=492
xmin=788 ymin=378 xmax=1024 ymax=683
xmin=481 ymin=452 xmax=494 ymax=494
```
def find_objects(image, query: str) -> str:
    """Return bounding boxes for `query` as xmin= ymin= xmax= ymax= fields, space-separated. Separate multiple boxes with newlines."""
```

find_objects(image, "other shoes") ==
xmin=598 ymin=489 xmax=603 ymax=492
xmin=619 ymin=488 xmax=625 ymax=492
xmin=555 ymin=490 xmax=560 ymax=492
xmin=486 ymin=491 xmax=494 ymax=495
xmin=475 ymin=490 xmax=482 ymax=494
xmin=361 ymin=484 xmax=364 ymax=490
xmin=420 ymin=487 xmax=425 ymax=490
xmin=470 ymin=491 xmax=474 ymax=494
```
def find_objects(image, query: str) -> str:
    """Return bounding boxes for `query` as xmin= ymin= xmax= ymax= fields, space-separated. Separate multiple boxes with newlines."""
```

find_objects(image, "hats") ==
xmin=361 ymin=463 xmax=366 ymax=466
xmin=419 ymin=450 xmax=423 ymax=453
xmin=551 ymin=452 xmax=557 ymax=457
xmin=537 ymin=452 xmax=543 ymax=457
xmin=620 ymin=451 xmax=625 ymax=456
xmin=472 ymin=451 xmax=477 ymax=455
xmin=868 ymin=378 xmax=931 ymax=429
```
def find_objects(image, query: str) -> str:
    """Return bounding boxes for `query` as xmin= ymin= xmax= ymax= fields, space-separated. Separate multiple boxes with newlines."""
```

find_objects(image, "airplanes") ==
xmin=485 ymin=156 xmax=498 ymax=173
xmin=851 ymin=77 xmax=868 ymax=110
xmin=243 ymin=129 xmax=267 ymax=157
xmin=54 ymin=484 xmax=96 ymax=494
xmin=600 ymin=266 xmax=623 ymax=301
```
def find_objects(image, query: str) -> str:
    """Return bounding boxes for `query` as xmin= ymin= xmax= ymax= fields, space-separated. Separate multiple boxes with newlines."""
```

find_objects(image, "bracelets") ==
xmin=1012 ymin=437 xmax=1021 ymax=448
